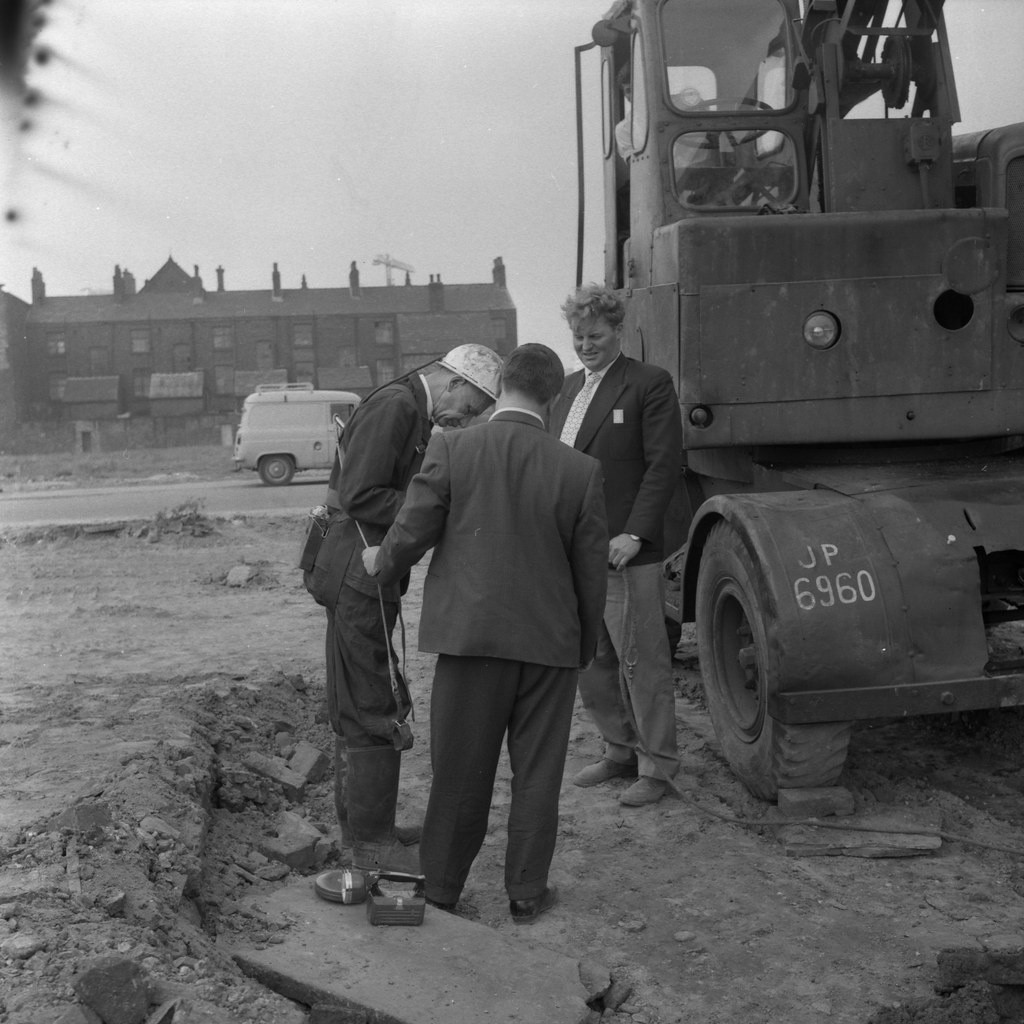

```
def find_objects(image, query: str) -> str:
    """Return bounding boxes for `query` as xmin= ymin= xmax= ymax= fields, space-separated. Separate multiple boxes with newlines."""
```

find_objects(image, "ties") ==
xmin=559 ymin=372 xmax=601 ymax=447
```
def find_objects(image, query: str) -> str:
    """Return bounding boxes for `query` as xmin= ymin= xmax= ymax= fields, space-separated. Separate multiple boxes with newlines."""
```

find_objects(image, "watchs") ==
xmin=630 ymin=533 xmax=639 ymax=541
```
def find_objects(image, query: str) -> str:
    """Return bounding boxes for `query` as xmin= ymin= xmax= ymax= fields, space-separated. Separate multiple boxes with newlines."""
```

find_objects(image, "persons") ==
xmin=362 ymin=343 xmax=609 ymax=922
xmin=299 ymin=344 xmax=502 ymax=876
xmin=545 ymin=282 xmax=694 ymax=805
xmin=615 ymin=61 xmax=795 ymax=206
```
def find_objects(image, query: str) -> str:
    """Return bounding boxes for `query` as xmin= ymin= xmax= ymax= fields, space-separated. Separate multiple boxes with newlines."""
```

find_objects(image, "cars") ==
xmin=229 ymin=382 xmax=364 ymax=490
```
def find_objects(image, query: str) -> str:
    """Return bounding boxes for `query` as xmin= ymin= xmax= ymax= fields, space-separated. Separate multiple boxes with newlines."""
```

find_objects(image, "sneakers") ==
xmin=618 ymin=777 xmax=672 ymax=805
xmin=572 ymin=760 xmax=627 ymax=787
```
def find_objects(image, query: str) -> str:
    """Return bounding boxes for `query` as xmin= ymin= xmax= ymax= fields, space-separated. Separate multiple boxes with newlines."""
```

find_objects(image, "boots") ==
xmin=343 ymin=745 xmax=423 ymax=881
xmin=333 ymin=735 xmax=422 ymax=848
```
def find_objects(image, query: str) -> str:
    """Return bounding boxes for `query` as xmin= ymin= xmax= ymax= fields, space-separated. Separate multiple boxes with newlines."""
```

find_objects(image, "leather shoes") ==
xmin=509 ymin=881 xmax=558 ymax=921
xmin=412 ymin=879 xmax=456 ymax=912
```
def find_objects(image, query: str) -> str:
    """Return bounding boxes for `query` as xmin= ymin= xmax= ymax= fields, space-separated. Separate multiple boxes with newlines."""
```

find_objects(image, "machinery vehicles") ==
xmin=559 ymin=0 xmax=1024 ymax=804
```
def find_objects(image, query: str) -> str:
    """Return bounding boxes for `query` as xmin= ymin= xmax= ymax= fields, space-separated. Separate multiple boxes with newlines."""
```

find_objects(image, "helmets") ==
xmin=436 ymin=344 xmax=504 ymax=400
